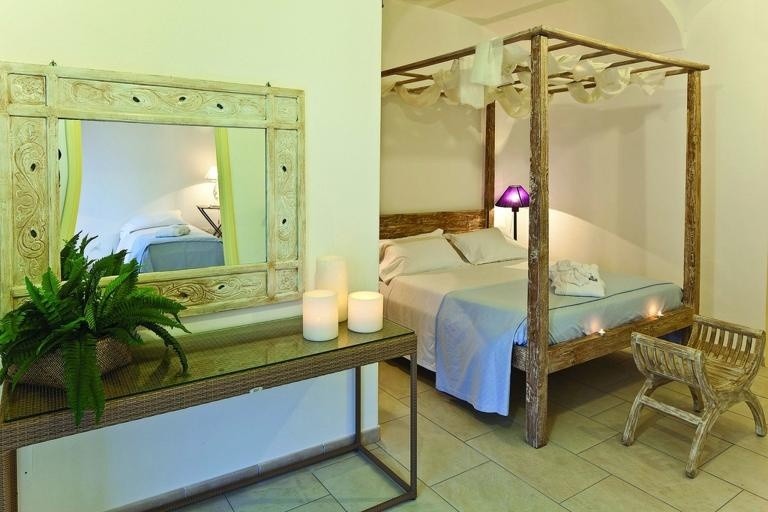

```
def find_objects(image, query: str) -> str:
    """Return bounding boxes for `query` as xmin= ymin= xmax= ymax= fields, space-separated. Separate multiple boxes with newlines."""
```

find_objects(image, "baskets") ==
xmin=2 ymin=333 xmax=133 ymax=390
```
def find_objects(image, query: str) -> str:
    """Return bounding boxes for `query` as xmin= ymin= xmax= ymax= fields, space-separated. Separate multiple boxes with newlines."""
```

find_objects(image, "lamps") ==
xmin=204 ymin=166 xmax=219 ymax=208
xmin=299 ymin=289 xmax=341 ymax=342
xmin=315 ymin=254 xmax=350 ymax=325
xmin=347 ymin=290 xmax=385 ymax=334
xmin=494 ymin=184 xmax=530 ymax=241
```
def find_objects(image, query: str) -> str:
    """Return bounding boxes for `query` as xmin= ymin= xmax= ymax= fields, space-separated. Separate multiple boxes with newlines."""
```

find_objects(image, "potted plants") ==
xmin=0 ymin=227 xmax=193 ymax=426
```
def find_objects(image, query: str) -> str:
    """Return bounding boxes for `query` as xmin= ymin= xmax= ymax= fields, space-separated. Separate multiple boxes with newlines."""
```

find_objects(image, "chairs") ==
xmin=621 ymin=312 xmax=766 ymax=480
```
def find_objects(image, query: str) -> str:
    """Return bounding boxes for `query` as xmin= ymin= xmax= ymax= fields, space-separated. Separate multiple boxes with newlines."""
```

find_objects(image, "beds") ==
xmin=380 ymin=26 xmax=715 ymax=451
xmin=115 ymin=210 xmax=225 ymax=273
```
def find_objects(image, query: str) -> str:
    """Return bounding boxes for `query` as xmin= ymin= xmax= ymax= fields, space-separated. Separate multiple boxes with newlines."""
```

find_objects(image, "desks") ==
xmin=0 ymin=314 xmax=417 ymax=512
xmin=193 ymin=203 xmax=222 ymax=239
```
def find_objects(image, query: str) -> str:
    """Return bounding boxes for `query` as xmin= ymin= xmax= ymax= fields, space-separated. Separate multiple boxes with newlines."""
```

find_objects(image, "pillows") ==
xmin=443 ymin=226 xmax=528 ymax=264
xmin=380 ymin=227 xmax=465 ymax=285
xmin=122 ymin=209 xmax=189 ymax=234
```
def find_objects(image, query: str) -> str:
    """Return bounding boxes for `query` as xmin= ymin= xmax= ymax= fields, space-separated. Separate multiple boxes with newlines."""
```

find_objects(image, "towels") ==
xmin=555 ymin=263 xmax=608 ymax=299
xmin=548 ymin=258 xmax=594 ymax=288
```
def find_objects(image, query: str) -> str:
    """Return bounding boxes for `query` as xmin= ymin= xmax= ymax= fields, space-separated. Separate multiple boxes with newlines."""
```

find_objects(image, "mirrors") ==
xmin=1 ymin=62 xmax=306 ymax=327
